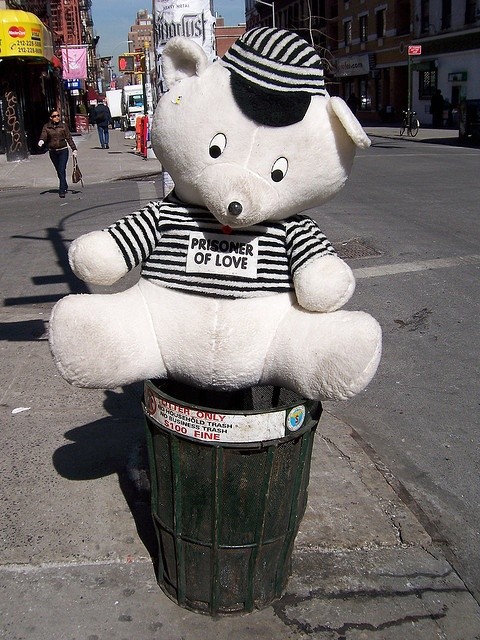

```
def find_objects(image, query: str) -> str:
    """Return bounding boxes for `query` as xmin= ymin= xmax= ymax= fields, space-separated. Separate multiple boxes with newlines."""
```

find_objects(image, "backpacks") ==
xmin=91 ymin=108 xmax=107 ymax=124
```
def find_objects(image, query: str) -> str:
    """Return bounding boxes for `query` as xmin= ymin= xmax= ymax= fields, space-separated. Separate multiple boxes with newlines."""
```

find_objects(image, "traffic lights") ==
xmin=118 ymin=56 xmax=134 ymax=71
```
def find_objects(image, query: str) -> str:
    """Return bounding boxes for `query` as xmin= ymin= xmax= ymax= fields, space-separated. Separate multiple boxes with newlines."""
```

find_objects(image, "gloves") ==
xmin=109 ymin=119 xmax=112 ymax=125
xmin=91 ymin=122 xmax=95 ymax=127
xmin=38 ymin=140 xmax=44 ymax=147
xmin=72 ymin=150 xmax=78 ymax=158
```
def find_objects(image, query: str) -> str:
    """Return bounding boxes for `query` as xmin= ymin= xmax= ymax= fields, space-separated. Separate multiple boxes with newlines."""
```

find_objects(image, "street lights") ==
xmin=110 ymin=40 xmax=133 ymax=81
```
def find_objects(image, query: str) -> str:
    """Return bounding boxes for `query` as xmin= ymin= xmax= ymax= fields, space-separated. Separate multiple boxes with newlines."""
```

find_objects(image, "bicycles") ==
xmin=400 ymin=111 xmax=420 ymax=137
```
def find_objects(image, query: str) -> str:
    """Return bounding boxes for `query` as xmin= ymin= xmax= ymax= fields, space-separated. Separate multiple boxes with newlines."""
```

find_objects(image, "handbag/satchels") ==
xmin=71 ymin=155 xmax=82 ymax=185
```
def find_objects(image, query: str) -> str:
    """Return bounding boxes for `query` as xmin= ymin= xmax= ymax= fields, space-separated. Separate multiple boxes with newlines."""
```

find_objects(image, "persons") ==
xmin=38 ymin=110 xmax=78 ymax=198
xmin=429 ymin=89 xmax=445 ymax=129
xmin=91 ymin=97 xmax=111 ymax=149
xmin=129 ymin=113 xmax=136 ymax=122
xmin=120 ymin=102 xmax=129 ymax=131
xmin=346 ymin=93 xmax=357 ymax=114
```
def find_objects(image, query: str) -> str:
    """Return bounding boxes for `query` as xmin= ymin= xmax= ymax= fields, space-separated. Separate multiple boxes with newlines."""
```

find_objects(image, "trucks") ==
xmin=121 ymin=84 xmax=144 ymax=131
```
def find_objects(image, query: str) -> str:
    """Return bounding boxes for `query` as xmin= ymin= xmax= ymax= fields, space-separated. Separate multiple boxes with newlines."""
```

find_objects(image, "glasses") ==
xmin=51 ymin=114 xmax=60 ymax=118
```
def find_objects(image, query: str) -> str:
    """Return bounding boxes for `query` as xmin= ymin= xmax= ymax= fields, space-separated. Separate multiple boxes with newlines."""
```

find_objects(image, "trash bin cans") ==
xmin=142 ymin=380 xmax=324 ymax=618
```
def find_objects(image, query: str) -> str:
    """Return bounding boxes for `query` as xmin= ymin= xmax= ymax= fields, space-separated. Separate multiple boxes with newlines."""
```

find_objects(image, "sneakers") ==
xmin=102 ymin=145 xmax=105 ymax=149
xmin=58 ymin=190 xmax=66 ymax=200
xmin=106 ymin=144 xmax=110 ymax=148
